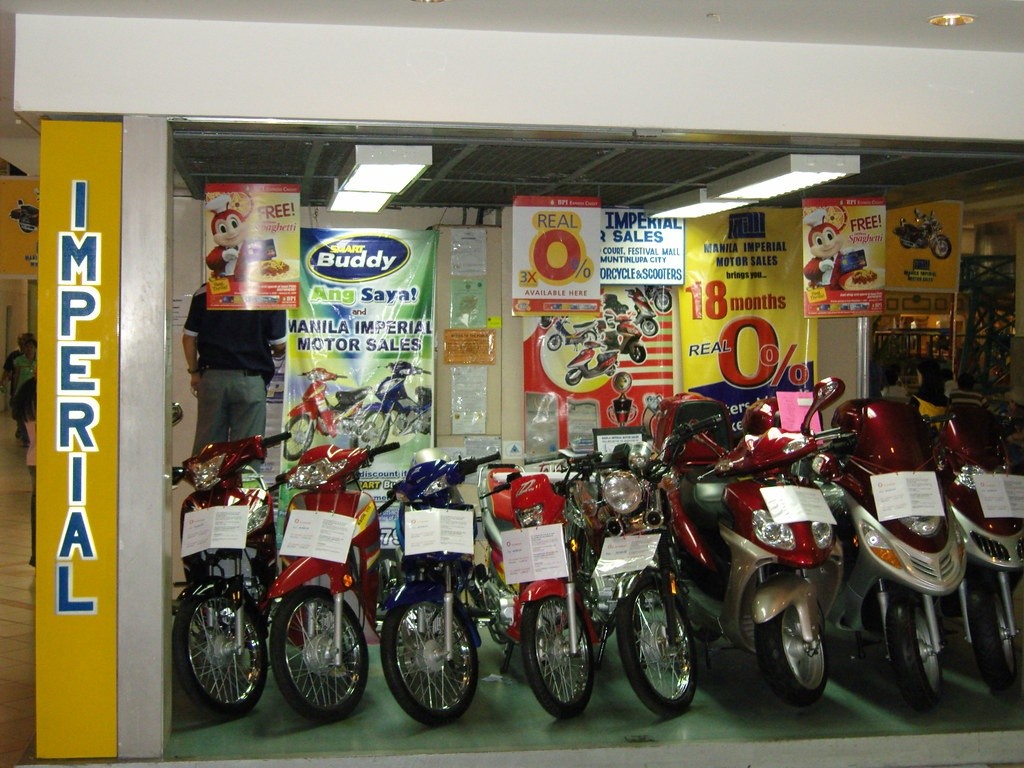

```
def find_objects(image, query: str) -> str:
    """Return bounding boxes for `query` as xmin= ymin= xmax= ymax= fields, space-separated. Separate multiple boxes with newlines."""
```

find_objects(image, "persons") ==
xmin=880 ymin=358 xmax=1024 ymax=476
xmin=183 ymin=282 xmax=289 ymax=476
xmin=0 ymin=331 xmax=37 ymax=567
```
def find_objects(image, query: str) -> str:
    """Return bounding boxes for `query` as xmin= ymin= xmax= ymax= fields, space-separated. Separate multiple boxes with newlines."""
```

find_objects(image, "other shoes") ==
xmin=16 ymin=427 xmax=22 ymax=439
xmin=24 ymin=439 xmax=30 ymax=447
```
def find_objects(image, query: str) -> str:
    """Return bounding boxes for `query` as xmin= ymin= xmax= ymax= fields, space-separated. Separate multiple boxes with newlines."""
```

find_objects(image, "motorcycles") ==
xmin=280 ymin=368 xmax=375 ymax=461
xmin=794 ymin=354 xmax=1024 ymax=716
xmin=171 ymin=430 xmax=293 ymax=722
xmin=895 ymin=208 xmax=952 ymax=260
xmin=552 ymin=414 xmax=723 ymax=718
xmin=375 ymin=450 xmax=502 ymax=727
xmin=540 ymin=285 xmax=673 ymax=387
xmin=650 ymin=377 xmax=858 ymax=706
xmin=469 ymin=451 xmax=616 ymax=721
xmin=261 ymin=441 xmax=400 ymax=724
xmin=347 ymin=360 xmax=432 ymax=451
